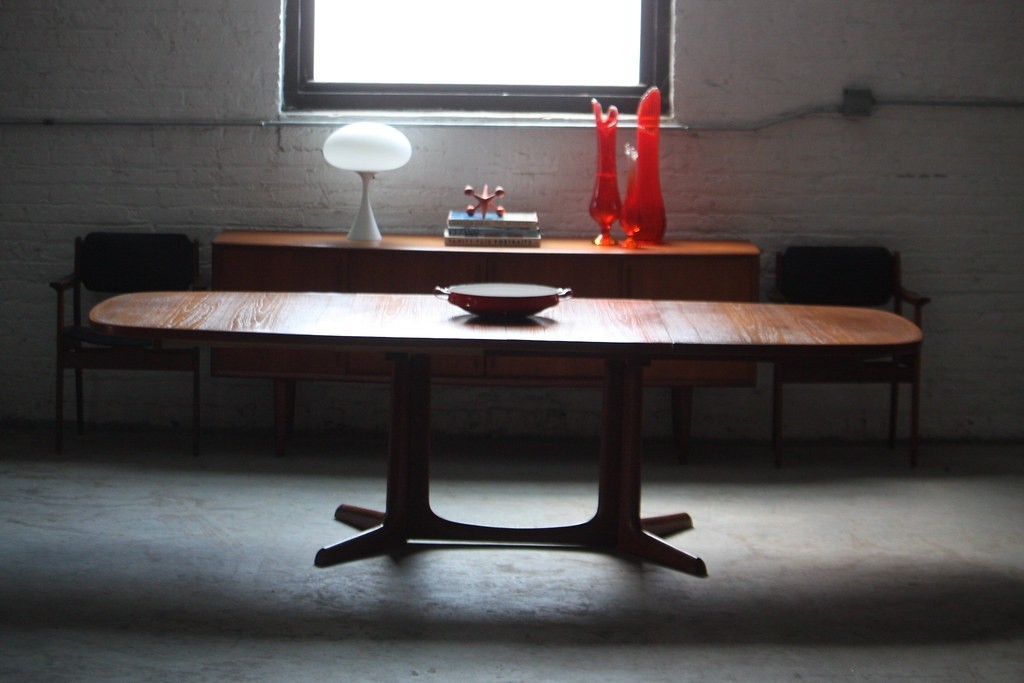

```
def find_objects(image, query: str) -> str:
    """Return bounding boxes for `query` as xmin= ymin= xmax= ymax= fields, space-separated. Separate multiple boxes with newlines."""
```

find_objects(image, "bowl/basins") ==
xmin=431 ymin=282 xmax=574 ymax=321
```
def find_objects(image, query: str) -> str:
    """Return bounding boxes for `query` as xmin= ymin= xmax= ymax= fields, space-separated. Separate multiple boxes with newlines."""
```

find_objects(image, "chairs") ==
xmin=49 ymin=232 xmax=200 ymax=460
xmin=764 ymin=246 xmax=932 ymax=469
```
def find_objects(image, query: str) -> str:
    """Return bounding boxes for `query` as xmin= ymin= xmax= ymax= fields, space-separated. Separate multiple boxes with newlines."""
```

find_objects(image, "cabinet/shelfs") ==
xmin=209 ymin=231 xmax=761 ymax=466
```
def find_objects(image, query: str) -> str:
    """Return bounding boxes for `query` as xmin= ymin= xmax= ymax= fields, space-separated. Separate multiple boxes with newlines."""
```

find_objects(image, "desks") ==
xmin=87 ymin=292 xmax=922 ymax=578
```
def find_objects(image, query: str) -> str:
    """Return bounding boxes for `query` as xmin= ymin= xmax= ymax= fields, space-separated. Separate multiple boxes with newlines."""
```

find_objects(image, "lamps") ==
xmin=322 ymin=120 xmax=412 ymax=240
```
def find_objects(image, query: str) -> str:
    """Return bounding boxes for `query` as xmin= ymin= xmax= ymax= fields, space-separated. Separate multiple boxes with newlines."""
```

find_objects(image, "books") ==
xmin=443 ymin=210 xmax=542 ymax=247
